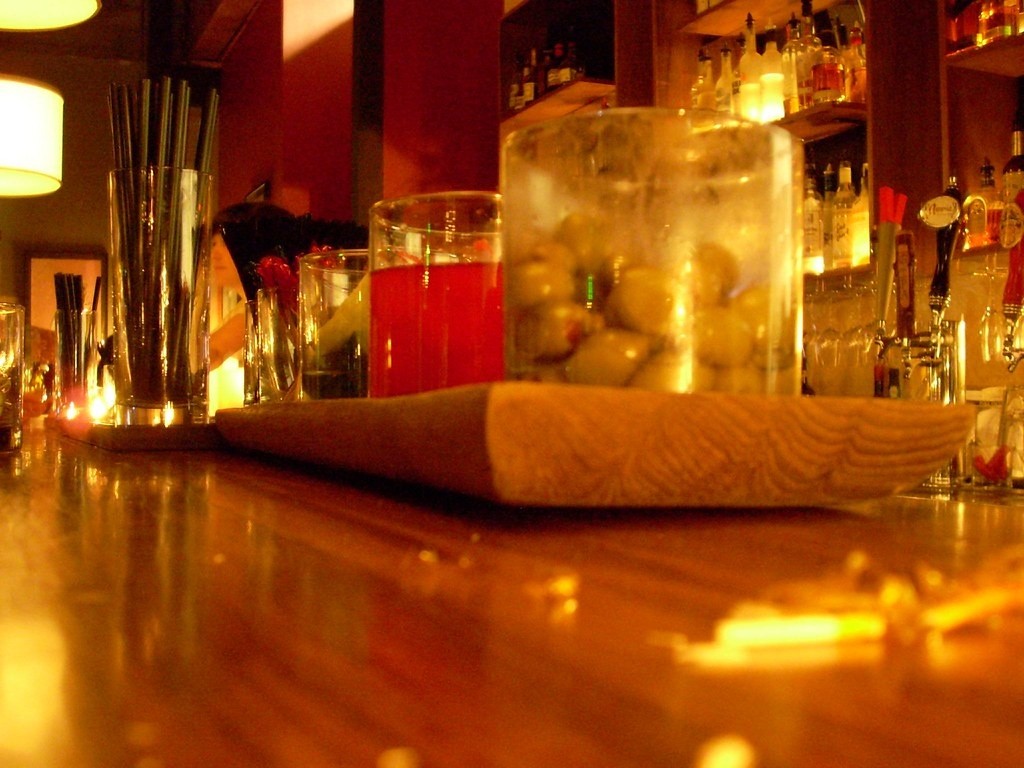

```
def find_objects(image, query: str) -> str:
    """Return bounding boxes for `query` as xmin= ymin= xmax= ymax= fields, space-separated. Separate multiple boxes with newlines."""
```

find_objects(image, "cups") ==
xmin=502 ymin=107 xmax=805 ymax=393
xmin=245 ymin=302 xmax=257 ymax=406
xmin=0 ymin=303 xmax=25 ymax=453
xmin=368 ymin=191 xmax=505 ymax=396
xmin=108 ymin=167 xmax=216 ymax=429
xmin=298 ymin=249 xmax=368 ymax=397
xmin=52 ymin=309 xmax=97 ymax=416
xmin=258 ymin=288 xmax=301 ymax=403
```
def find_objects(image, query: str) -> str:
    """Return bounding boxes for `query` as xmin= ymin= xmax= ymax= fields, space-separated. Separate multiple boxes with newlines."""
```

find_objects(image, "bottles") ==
xmin=689 ymin=1 xmax=866 ymax=123
xmin=502 ymin=36 xmax=599 ymax=112
xmin=802 ymin=161 xmax=869 ymax=278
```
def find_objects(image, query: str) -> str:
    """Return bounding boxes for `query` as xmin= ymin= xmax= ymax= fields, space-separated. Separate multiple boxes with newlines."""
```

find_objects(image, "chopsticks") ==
xmin=54 ymin=271 xmax=84 ymax=405
xmin=104 ymin=76 xmax=219 ymax=395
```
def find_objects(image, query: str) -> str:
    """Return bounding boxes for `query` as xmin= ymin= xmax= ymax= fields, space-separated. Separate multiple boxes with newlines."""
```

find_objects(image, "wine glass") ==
xmin=973 ymin=268 xmax=1008 ymax=363
xmin=810 ymin=280 xmax=884 ymax=403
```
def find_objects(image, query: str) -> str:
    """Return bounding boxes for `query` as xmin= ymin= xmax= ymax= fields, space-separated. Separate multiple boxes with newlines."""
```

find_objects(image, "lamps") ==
xmin=0 ymin=75 xmax=66 ymax=200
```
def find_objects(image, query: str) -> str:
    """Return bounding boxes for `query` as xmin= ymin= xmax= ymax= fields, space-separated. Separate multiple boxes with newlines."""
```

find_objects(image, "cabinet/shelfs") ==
xmin=497 ymin=0 xmax=1023 ymax=279
